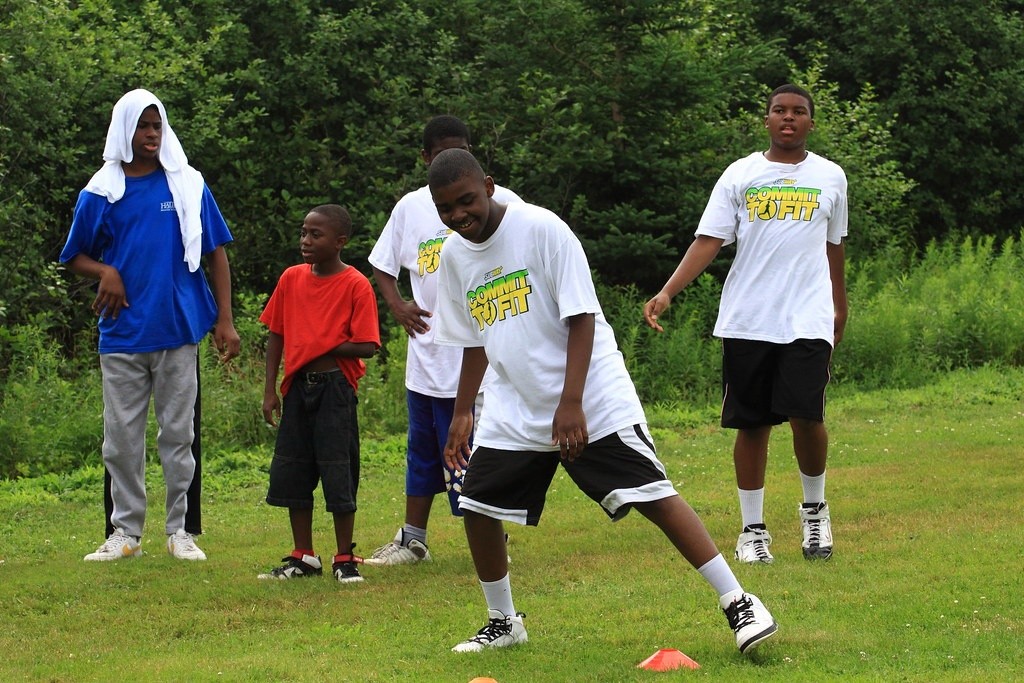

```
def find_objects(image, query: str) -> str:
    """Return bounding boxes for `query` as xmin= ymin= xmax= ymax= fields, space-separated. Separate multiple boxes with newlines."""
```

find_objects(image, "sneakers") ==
xmin=364 ymin=527 xmax=433 ymax=568
xmin=332 ymin=542 xmax=364 ymax=584
xmin=503 ymin=532 xmax=512 ymax=564
xmin=734 ymin=524 xmax=774 ymax=565
xmin=450 ymin=608 xmax=528 ymax=653
xmin=718 ymin=589 xmax=778 ymax=655
xmin=798 ymin=499 xmax=833 ymax=563
xmin=84 ymin=527 xmax=142 ymax=564
xmin=258 ymin=548 xmax=323 ymax=581
xmin=166 ymin=528 xmax=207 ymax=560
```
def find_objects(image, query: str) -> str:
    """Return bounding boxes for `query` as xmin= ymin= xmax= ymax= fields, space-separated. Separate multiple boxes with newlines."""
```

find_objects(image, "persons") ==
xmin=256 ymin=205 xmax=381 ymax=582
xmin=426 ymin=148 xmax=779 ymax=654
xmin=58 ymin=89 xmax=241 ymax=562
xmin=644 ymin=84 xmax=849 ymax=569
xmin=360 ymin=115 xmax=527 ymax=565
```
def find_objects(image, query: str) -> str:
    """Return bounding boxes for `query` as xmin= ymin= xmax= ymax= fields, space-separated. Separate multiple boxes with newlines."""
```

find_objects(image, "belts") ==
xmin=293 ymin=372 xmax=347 ymax=385
